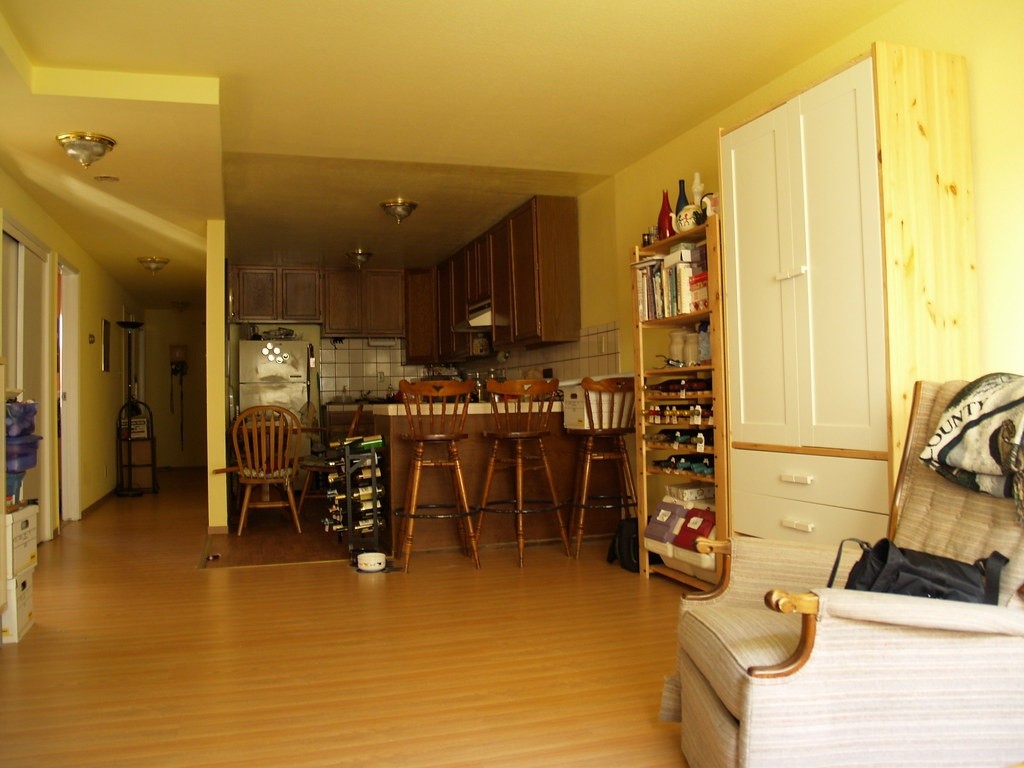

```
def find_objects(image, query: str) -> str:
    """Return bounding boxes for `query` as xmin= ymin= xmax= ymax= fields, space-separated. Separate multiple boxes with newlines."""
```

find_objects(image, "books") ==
xmin=636 ymin=244 xmax=708 ymax=323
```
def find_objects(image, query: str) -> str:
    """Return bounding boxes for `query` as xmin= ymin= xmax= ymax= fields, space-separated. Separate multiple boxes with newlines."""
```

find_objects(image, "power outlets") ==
xmin=377 ymin=372 xmax=385 ymax=383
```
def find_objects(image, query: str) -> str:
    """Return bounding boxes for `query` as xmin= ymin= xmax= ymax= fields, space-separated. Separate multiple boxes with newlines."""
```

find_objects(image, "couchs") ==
xmin=659 ymin=371 xmax=1024 ymax=768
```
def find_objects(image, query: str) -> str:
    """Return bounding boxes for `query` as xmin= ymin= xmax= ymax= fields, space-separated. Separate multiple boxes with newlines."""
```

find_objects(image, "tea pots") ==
xmin=693 ymin=193 xmax=715 ymax=225
xmin=461 ymin=387 xmax=479 ymax=402
xmin=669 ymin=201 xmax=704 ymax=234
xmin=702 ymin=197 xmax=720 ymax=217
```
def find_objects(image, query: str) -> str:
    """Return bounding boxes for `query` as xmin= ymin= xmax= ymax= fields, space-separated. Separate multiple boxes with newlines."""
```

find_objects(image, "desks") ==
xmin=229 ymin=423 xmax=323 ymax=524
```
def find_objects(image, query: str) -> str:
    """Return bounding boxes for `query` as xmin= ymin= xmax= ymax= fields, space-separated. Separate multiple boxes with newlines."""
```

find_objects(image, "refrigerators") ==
xmin=239 ymin=339 xmax=311 ymax=491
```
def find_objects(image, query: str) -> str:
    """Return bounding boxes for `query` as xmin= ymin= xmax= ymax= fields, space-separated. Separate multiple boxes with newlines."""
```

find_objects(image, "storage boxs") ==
xmin=6 ymin=504 xmax=41 ymax=580
xmin=664 ymin=250 xmax=701 ymax=267
xmin=0 ymin=568 xmax=37 ymax=645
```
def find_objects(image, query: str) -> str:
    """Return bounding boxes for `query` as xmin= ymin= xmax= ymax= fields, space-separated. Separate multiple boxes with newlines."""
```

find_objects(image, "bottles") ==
xmin=692 ymin=172 xmax=704 ymax=206
xmin=641 ymin=378 xmax=713 ymax=476
xmin=472 ymin=372 xmax=486 ymax=399
xmin=658 ymin=189 xmax=675 ymax=240
xmin=472 ymin=333 xmax=490 ymax=355
xmin=669 ymin=332 xmax=698 ymax=366
xmin=676 ymin=180 xmax=688 ymax=217
xmin=321 ymin=434 xmax=387 ymax=533
xmin=421 ymin=364 xmax=457 ymax=376
xmin=490 ymin=368 xmax=507 ymax=403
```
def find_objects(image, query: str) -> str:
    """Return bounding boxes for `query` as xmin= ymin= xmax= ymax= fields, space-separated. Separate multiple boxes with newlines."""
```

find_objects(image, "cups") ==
xmin=649 ymin=225 xmax=658 ymax=244
xmin=643 ymin=234 xmax=650 ymax=247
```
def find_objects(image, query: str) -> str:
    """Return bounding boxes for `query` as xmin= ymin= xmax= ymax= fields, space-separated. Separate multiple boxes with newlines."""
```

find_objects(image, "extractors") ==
xmin=449 ymin=307 xmax=492 ymax=332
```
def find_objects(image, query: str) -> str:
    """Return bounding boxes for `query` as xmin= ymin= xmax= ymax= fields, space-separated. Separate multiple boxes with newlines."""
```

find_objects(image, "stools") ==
xmin=567 ymin=377 xmax=640 ymax=559
xmin=469 ymin=379 xmax=570 ymax=566
xmin=397 ymin=379 xmax=482 ymax=573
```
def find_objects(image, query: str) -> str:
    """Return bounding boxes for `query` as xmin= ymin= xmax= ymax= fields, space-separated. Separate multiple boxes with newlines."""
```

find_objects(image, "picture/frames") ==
xmin=102 ymin=319 xmax=110 ymax=372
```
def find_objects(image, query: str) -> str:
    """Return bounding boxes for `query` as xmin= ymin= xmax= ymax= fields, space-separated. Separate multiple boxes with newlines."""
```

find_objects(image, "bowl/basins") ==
xmin=335 ymin=395 xmax=352 ymax=403
xmin=4 ymin=388 xmax=23 ymax=400
xmin=349 ymin=547 xmax=387 ymax=572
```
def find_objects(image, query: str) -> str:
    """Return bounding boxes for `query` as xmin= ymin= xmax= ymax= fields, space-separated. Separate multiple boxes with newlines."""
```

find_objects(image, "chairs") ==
xmin=298 ymin=405 xmax=365 ymax=516
xmin=231 ymin=406 xmax=304 ymax=536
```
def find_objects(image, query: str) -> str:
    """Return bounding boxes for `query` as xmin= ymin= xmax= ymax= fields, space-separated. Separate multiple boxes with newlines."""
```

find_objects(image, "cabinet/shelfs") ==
xmin=118 ymin=400 xmax=161 ymax=494
xmin=328 ymin=435 xmax=388 ymax=559
xmin=629 ymin=213 xmax=731 ymax=591
xmin=714 ymin=42 xmax=975 ymax=582
xmin=232 ymin=195 xmax=582 ymax=366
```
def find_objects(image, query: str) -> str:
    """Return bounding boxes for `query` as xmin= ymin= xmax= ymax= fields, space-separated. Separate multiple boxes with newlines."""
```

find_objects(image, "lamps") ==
xmin=116 ymin=321 xmax=145 ymax=497
xmin=379 ymin=200 xmax=419 ymax=226
xmin=170 ymin=300 xmax=191 ymax=312
xmin=137 ymin=256 xmax=169 ymax=279
xmin=55 ymin=131 xmax=117 ymax=168
xmin=169 ymin=345 xmax=188 ymax=452
xmin=347 ymin=248 xmax=373 ymax=269
